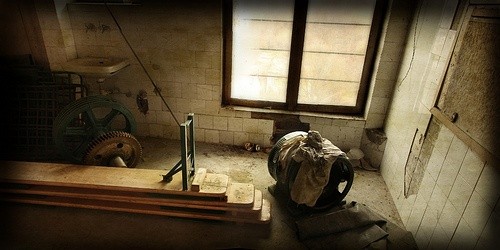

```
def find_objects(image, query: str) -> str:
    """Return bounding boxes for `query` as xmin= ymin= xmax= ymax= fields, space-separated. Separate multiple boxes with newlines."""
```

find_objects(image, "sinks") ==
xmin=64 ymin=56 xmax=129 ymax=76
